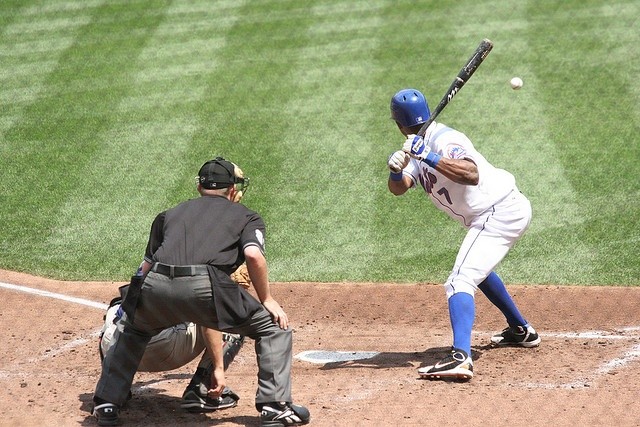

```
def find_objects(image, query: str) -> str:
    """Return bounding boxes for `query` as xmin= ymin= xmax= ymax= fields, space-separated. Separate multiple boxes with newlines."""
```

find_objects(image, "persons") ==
xmin=89 ymin=157 xmax=310 ymax=427
xmin=387 ymin=88 xmax=542 ymax=382
xmin=92 ymin=262 xmax=252 ymax=413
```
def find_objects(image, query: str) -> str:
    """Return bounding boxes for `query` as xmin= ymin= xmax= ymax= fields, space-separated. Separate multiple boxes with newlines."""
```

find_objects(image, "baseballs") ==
xmin=510 ymin=77 xmax=523 ymax=90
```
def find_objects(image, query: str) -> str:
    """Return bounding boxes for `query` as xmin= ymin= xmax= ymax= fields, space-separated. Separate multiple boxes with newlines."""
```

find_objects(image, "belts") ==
xmin=149 ymin=262 xmax=210 ymax=277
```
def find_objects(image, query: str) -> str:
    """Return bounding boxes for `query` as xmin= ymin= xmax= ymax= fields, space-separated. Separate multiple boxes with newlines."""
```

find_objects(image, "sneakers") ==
xmin=90 ymin=401 xmax=120 ymax=426
xmin=180 ymin=382 xmax=239 ymax=413
xmin=418 ymin=346 xmax=473 ymax=379
xmin=490 ymin=318 xmax=542 ymax=348
xmin=260 ymin=402 xmax=310 ymax=427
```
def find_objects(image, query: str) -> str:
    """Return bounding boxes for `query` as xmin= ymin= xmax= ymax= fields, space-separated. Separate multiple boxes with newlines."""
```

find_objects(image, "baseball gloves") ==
xmin=229 ymin=262 xmax=251 ymax=291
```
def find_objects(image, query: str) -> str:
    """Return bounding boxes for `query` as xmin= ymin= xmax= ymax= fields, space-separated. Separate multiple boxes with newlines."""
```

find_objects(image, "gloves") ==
xmin=401 ymin=134 xmax=432 ymax=167
xmin=387 ymin=150 xmax=411 ymax=175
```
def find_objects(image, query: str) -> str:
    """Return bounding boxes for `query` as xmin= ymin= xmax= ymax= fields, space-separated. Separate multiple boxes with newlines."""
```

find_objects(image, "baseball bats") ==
xmin=389 ymin=38 xmax=495 ymax=173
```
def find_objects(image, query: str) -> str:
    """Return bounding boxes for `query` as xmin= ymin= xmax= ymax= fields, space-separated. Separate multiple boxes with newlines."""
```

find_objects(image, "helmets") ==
xmin=389 ymin=89 xmax=431 ymax=129
xmin=194 ymin=157 xmax=250 ymax=203
